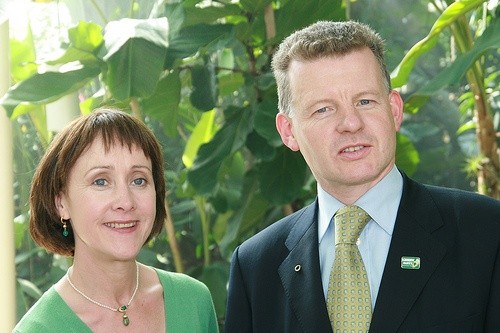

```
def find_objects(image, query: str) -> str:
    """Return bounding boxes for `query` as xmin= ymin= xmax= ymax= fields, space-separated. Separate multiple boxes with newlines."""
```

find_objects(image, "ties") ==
xmin=326 ymin=204 xmax=372 ymax=333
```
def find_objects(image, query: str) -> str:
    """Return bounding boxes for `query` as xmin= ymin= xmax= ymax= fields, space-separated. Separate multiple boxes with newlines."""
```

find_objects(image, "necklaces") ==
xmin=66 ymin=261 xmax=139 ymax=326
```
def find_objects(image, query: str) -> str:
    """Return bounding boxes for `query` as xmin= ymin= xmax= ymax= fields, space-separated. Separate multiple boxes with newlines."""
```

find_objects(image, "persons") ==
xmin=7 ymin=108 xmax=220 ymax=333
xmin=224 ymin=20 xmax=500 ymax=333
